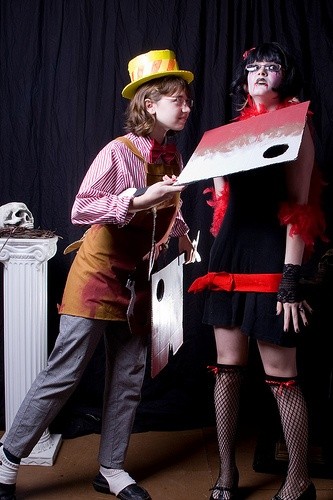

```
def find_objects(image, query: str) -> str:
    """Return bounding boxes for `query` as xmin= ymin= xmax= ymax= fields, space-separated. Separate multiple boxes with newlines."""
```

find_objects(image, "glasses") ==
xmin=244 ymin=63 xmax=284 ymax=75
xmin=159 ymin=97 xmax=193 ymax=107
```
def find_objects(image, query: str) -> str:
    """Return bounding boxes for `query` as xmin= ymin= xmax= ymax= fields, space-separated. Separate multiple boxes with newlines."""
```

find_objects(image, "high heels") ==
xmin=271 ymin=482 xmax=316 ymax=500
xmin=209 ymin=465 xmax=239 ymax=500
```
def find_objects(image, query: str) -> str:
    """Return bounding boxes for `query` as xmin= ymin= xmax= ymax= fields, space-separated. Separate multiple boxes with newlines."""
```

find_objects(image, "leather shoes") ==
xmin=92 ymin=470 xmax=152 ymax=500
xmin=0 ymin=443 xmax=17 ymax=500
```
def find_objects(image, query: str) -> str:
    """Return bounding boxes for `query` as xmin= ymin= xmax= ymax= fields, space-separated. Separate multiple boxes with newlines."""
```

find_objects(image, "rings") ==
xmin=298 ymin=305 xmax=304 ymax=313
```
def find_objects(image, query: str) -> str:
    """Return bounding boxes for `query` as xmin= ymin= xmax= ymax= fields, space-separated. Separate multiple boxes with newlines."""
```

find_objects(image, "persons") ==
xmin=188 ymin=41 xmax=330 ymax=500
xmin=0 ymin=50 xmax=194 ymax=500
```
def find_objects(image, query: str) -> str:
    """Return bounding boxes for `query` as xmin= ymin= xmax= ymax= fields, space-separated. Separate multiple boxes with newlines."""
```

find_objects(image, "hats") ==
xmin=122 ymin=49 xmax=194 ymax=99
